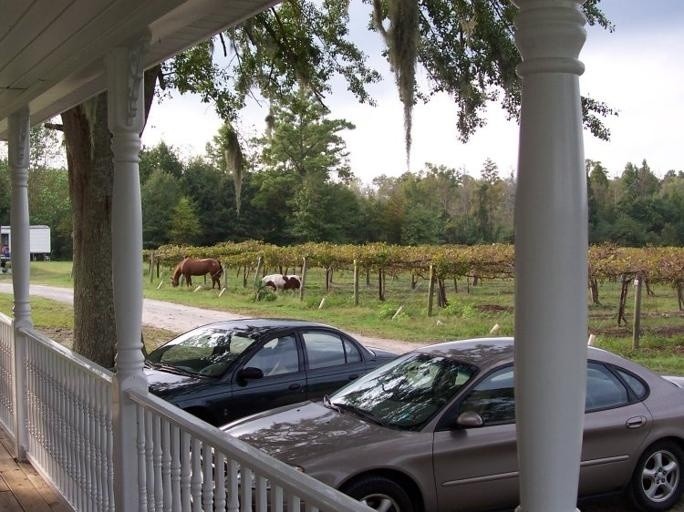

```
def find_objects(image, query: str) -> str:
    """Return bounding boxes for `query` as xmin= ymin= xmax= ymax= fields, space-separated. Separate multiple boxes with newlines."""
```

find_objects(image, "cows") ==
xmin=258 ymin=273 xmax=303 ymax=297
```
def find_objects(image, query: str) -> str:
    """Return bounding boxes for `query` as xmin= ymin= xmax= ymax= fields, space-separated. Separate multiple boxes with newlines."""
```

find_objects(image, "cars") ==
xmin=167 ymin=333 xmax=684 ymax=511
xmin=131 ymin=314 xmax=403 ymax=435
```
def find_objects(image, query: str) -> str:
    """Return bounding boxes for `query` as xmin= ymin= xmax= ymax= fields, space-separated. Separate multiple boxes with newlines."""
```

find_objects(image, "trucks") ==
xmin=1 ymin=223 xmax=53 ymax=262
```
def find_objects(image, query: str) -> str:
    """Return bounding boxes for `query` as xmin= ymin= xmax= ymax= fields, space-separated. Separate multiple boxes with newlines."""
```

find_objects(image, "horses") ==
xmin=170 ymin=257 xmax=225 ymax=290
